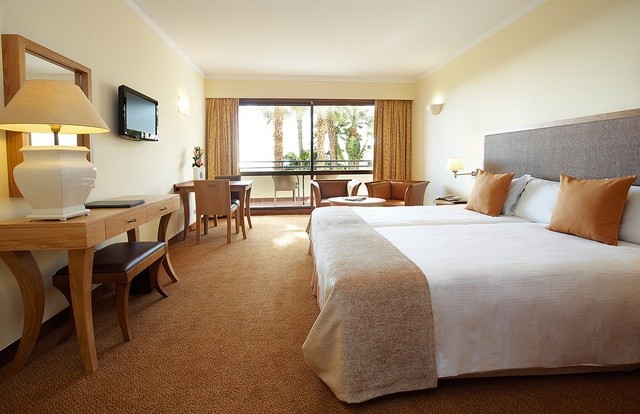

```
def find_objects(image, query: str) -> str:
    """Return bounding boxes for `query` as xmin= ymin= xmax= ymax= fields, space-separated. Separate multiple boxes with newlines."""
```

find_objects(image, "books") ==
xmin=84 ymin=201 xmax=144 ymax=209
xmin=345 ymin=197 xmax=366 ymax=201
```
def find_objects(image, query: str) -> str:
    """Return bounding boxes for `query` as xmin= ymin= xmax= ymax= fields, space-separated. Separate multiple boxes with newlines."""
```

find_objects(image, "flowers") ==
xmin=192 ymin=146 xmax=205 ymax=167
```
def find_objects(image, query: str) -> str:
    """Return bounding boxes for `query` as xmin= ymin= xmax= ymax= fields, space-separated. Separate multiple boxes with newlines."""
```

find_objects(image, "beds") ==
xmin=302 ymin=107 xmax=640 ymax=404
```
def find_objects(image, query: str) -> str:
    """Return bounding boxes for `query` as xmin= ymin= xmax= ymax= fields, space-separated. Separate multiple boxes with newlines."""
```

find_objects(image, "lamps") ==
xmin=0 ymin=79 xmax=111 ymax=221
xmin=447 ymin=156 xmax=477 ymax=179
xmin=427 ymin=104 xmax=443 ymax=115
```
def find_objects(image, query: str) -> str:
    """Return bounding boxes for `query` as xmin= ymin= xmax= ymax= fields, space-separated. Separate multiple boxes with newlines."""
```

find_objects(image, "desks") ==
xmin=0 ymin=195 xmax=181 ymax=376
xmin=174 ymin=180 xmax=255 ymax=241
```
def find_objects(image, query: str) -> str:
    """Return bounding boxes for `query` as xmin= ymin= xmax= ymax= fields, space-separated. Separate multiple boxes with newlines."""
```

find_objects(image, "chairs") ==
xmin=194 ymin=179 xmax=240 ymax=246
xmin=272 ymin=174 xmax=300 ymax=203
xmin=214 ymin=175 xmax=243 ymax=234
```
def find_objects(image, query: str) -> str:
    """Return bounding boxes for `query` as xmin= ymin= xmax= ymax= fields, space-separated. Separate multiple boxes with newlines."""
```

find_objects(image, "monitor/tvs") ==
xmin=118 ymin=84 xmax=159 ymax=142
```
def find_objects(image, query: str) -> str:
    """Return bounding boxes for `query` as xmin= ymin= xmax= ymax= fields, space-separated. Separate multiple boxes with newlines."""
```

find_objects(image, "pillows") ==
xmin=515 ymin=177 xmax=561 ymax=224
xmin=544 ymin=173 xmax=638 ymax=245
xmin=619 ymin=186 xmax=640 ymax=245
xmin=502 ymin=173 xmax=531 ymax=215
xmin=464 ymin=168 xmax=515 ymax=217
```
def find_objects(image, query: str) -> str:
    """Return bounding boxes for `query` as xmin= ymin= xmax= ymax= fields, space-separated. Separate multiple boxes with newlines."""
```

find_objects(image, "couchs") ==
xmin=364 ymin=179 xmax=430 ymax=206
xmin=310 ymin=177 xmax=362 ymax=209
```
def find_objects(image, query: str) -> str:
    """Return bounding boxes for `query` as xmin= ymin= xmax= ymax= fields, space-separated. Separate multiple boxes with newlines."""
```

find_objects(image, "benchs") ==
xmin=52 ymin=240 xmax=169 ymax=343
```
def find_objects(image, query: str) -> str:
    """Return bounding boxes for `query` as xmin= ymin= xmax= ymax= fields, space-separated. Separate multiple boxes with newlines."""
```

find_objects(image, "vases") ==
xmin=193 ymin=167 xmax=206 ymax=181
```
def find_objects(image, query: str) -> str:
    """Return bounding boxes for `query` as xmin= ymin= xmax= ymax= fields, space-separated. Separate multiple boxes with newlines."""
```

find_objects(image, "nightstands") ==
xmin=435 ymin=196 xmax=467 ymax=205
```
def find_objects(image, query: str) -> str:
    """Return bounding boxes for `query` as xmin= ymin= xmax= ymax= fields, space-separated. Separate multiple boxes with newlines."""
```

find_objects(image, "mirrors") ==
xmin=1 ymin=34 xmax=96 ymax=198
